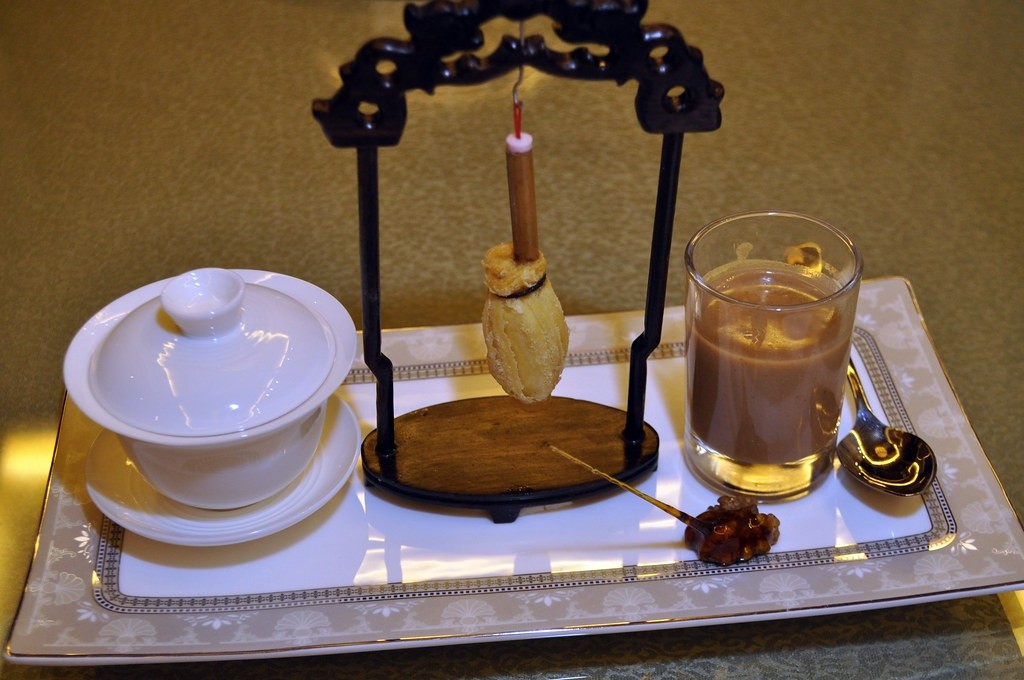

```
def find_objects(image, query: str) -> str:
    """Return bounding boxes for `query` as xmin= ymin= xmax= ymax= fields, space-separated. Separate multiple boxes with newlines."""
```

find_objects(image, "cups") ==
xmin=682 ymin=209 xmax=864 ymax=507
xmin=59 ymin=266 xmax=359 ymax=513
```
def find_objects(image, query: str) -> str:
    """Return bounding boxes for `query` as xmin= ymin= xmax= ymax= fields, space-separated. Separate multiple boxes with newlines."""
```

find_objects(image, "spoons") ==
xmin=834 ymin=352 xmax=937 ymax=499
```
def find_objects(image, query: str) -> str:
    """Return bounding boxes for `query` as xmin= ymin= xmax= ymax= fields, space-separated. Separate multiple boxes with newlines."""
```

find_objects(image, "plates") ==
xmin=3 ymin=275 xmax=1024 ymax=670
xmin=81 ymin=394 xmax=359 ymax=550
